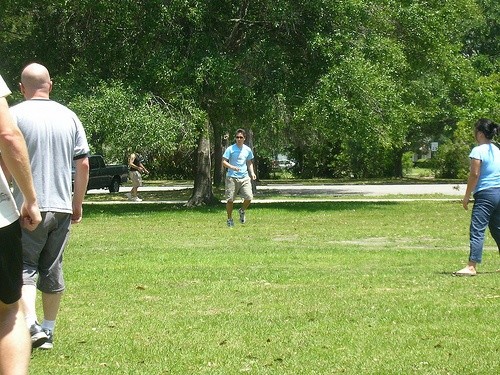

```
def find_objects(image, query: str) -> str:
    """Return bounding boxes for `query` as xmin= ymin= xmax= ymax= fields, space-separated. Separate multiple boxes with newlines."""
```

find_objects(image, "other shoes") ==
xmin=29 ymin=320 xmax=48 ymax=348
xmin=39 ymin=327 xmax=54 ymax=349
xmin=129 ymin=196 xmax=143 ymax=202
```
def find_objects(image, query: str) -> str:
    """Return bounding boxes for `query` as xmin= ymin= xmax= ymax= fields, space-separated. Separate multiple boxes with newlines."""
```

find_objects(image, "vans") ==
xmin=272 ymin=159 xmax=296 ymax=167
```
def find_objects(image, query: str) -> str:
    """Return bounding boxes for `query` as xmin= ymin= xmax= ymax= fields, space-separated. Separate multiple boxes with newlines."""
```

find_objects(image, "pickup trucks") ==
xmin=72 ymin=155 xmax=128 ymax=195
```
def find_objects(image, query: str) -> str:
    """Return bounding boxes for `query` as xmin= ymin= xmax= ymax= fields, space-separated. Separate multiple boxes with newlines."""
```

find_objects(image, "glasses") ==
xmin=235 ymin=136 xmax=244 ymax=139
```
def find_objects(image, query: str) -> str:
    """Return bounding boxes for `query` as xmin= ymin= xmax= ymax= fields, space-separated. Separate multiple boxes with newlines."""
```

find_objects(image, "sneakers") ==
xmin=226 ymin=217 xmax=234 ymax=228
xmin=238 ymin=208 xmax=246 ymax=224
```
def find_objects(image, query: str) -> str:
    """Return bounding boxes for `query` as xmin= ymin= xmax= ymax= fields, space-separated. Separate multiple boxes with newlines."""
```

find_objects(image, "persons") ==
xmin=453 ymin=117 xmax=500 ymax=275
xmin=8 ymin=61 xmax=91 ymax=349
xmin=125 ymin=147 xmax=149 ymax=202
xmin=222 ymin=128 xmax=256 ymax=228
xmin=0 ymin=73 xmax=41 ymax=375
